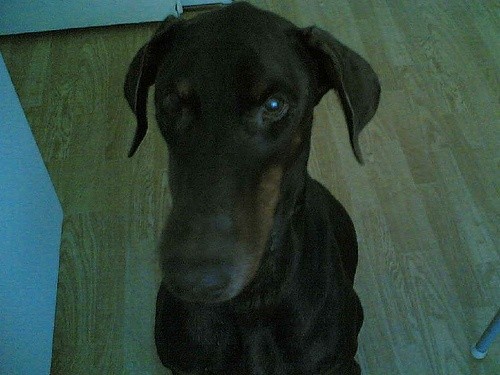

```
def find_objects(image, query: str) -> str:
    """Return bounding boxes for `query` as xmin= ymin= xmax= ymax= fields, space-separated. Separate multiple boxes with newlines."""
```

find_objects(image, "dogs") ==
xmin=121 ymin=1 xmax=380 ymax=375
